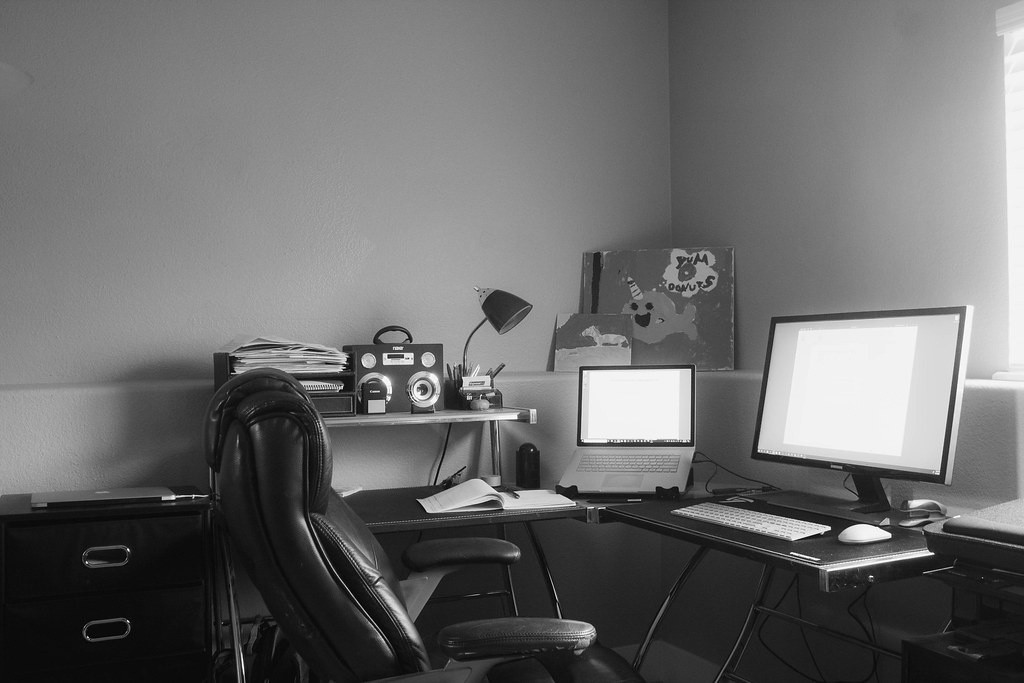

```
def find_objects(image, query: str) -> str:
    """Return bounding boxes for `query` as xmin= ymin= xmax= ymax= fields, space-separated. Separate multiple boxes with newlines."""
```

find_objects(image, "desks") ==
xmin=342 ymin=483 xmax=595 ymax=660
xmin=903 ymin=500 xmax=1023 ymax=628
xmin=319 ymin=408 xmax=538 ymax=483
xmin=606 ymin=491 xmax=960 ymax=683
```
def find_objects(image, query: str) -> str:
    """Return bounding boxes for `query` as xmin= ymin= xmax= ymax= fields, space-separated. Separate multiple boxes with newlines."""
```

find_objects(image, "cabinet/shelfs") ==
xmin=0 ymin=486 xmax=215 ymax=683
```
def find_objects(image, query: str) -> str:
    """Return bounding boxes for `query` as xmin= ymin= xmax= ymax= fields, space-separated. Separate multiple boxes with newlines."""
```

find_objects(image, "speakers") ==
xmin=362 ymin=381 xmax=386 ymax=414
xmin=342 ymin=340 xmax=443 ymax=415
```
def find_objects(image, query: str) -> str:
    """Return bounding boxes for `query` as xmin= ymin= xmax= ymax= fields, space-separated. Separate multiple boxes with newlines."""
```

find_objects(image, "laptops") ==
xmin=557 ymin=365 xmax=697 ymax=493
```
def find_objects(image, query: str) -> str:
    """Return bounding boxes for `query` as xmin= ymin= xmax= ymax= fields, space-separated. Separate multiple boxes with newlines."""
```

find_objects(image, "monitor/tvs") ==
xmin=752 ymin=303 xmax=974 ymax=523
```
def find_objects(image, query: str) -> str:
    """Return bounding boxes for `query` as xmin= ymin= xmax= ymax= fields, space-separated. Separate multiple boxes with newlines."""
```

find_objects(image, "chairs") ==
xmin=205 ymin=369 xmax=643 ymax=683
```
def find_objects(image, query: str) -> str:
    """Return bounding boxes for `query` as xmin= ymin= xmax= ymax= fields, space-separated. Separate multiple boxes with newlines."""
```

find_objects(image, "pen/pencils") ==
xmin=486 ymin=363 xmax=506 ymax=379
xmin=501 ymin=485 xmax=520 ymax=499
xmin=446 ymin=362 xmax=480 ymax=380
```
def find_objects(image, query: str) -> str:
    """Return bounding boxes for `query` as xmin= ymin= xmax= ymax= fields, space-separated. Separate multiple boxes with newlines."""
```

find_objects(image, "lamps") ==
xmin=458 ymin=286 xmax=532 ymax=408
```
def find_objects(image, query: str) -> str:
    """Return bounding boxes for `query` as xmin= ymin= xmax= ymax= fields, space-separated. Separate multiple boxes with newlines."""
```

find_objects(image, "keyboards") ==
xmin=671 ymin=500 xmax=831 ymax=541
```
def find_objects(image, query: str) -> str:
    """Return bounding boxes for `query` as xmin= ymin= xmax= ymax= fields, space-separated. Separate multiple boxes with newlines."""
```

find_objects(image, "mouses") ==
xmin=838 ymin=524 xmax=892 ymax=543
